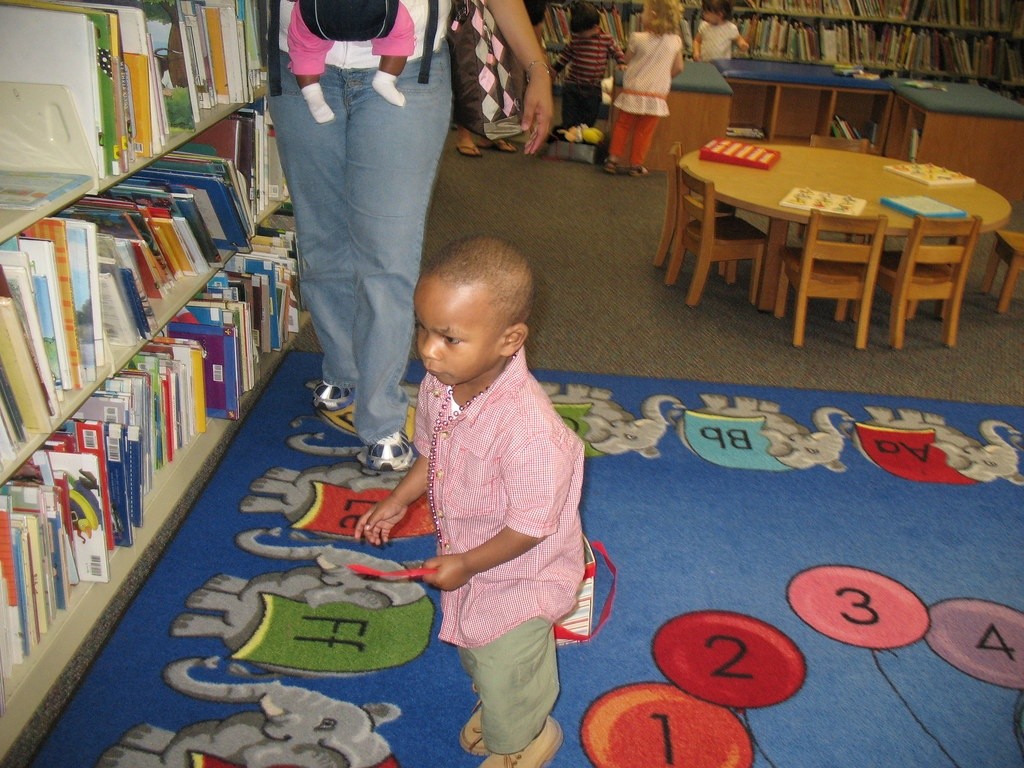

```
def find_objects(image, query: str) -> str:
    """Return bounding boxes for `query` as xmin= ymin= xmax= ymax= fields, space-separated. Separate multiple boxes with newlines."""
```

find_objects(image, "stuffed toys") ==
xmin=558 ymin=124 xmax=603 ymax=143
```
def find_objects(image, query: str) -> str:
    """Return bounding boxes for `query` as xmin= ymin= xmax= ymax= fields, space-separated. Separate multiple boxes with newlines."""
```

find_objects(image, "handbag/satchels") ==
xmin=446 ymin=0 xmax=527 ymax=139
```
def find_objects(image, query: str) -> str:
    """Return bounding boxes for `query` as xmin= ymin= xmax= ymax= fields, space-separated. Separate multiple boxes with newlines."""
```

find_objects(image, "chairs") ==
xmin=982 ymin=230 xmax=1024 ymax=315
xmin=663 ymin=164 xmax=767 ymax=309
xmin=797 ymin=136 xmax=871 ymax=244
xmin=774 ymin=208 xmax=888 ymax=351
xmin=652 ymin=144 xmax=738 ymax=275
xmin=853 ymin=215 xmax=982 ymax=350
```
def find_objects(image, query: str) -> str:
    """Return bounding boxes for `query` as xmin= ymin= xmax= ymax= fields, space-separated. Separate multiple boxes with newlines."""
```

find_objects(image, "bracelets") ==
xmin=526 ymin=61 xmax=551 ymax=83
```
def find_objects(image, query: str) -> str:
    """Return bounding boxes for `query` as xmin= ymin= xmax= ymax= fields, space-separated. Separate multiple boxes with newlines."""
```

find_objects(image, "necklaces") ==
xmin=425 ymin=354 xmax=515 ymax=549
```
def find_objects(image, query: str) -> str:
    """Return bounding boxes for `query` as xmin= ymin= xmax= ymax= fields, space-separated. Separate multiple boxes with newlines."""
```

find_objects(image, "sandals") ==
xmin=629 ymin=164 xmax=651 ymax=177
xmin=600 ymin=159 xmax=619 ymax=173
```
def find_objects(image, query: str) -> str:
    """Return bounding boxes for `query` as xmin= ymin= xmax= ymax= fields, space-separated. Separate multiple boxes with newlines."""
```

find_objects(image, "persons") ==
xmin=603 ymin=0 xmax=685 ymax=176
xmin=445 ymin=0 xmax=554 ymax=158
xmin=354 ymin=234 xmax=587 ymax=768
xmin=287 ymin=0 xmax=415 ymax=123
xmin=693 ymin=0 xmax=750 ymax=63
xmin=549 ymin=3 xmax=629 ymax=139
xmin=265 ymin=0 xmax=451 ymax=472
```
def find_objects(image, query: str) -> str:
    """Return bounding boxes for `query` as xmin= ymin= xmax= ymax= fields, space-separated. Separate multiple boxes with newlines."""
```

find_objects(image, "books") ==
xmin=884 ymin=162 xmax=977 ymax=188
xmin=0 ymin=0 xmax=311 ymax=708
xmin=832 ymin=115 xmax=877 ymax=145
xmin=881 ymin=194 xmax=966 ymax=218
xmin=537 ymin=0 xmax=1024 ymax=88
xmin=780 ymin=187 xmax=868 ymax=217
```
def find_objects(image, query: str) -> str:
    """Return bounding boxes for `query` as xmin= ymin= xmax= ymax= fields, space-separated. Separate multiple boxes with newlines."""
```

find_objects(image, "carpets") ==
xmin=29 ymin=350 xmax=1024 ymax=768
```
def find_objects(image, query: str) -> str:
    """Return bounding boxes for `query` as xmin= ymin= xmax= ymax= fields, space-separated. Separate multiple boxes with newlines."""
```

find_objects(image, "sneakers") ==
xmin=366 ymin=424 xmax=413 ymax=471
xmin=312 ymin=380 xmax=356 ymax=410
xmin=478 ymin=713 xmax=565 ymax=768
xmin=461 ymin=699 xmax=492 ymax=757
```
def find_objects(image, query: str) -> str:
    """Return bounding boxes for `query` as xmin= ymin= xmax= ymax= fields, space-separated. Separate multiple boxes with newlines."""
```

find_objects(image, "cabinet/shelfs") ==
xmin=882 ymin=77 xmax=1024 ymax=205
xmin=539 ymin=0 xmax=1024 ymax=107
xmin=0 ymin=0 xmax=310 ymax=768
xmin=609 ymin=61 xmax=734 ymax=172
xmin=712 ymin=57 xmax=892 ymax=157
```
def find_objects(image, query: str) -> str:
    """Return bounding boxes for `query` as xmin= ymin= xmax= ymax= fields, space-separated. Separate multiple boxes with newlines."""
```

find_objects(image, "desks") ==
xmin=679 ymin=144 xmax=1012 ymax=320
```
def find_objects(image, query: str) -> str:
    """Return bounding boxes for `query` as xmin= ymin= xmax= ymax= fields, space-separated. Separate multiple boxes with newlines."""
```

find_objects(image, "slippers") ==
xmin=456 ymin=141 xmax=482 ymax=159
xmin=477 ymin=138 xmax=518 ymax=153
